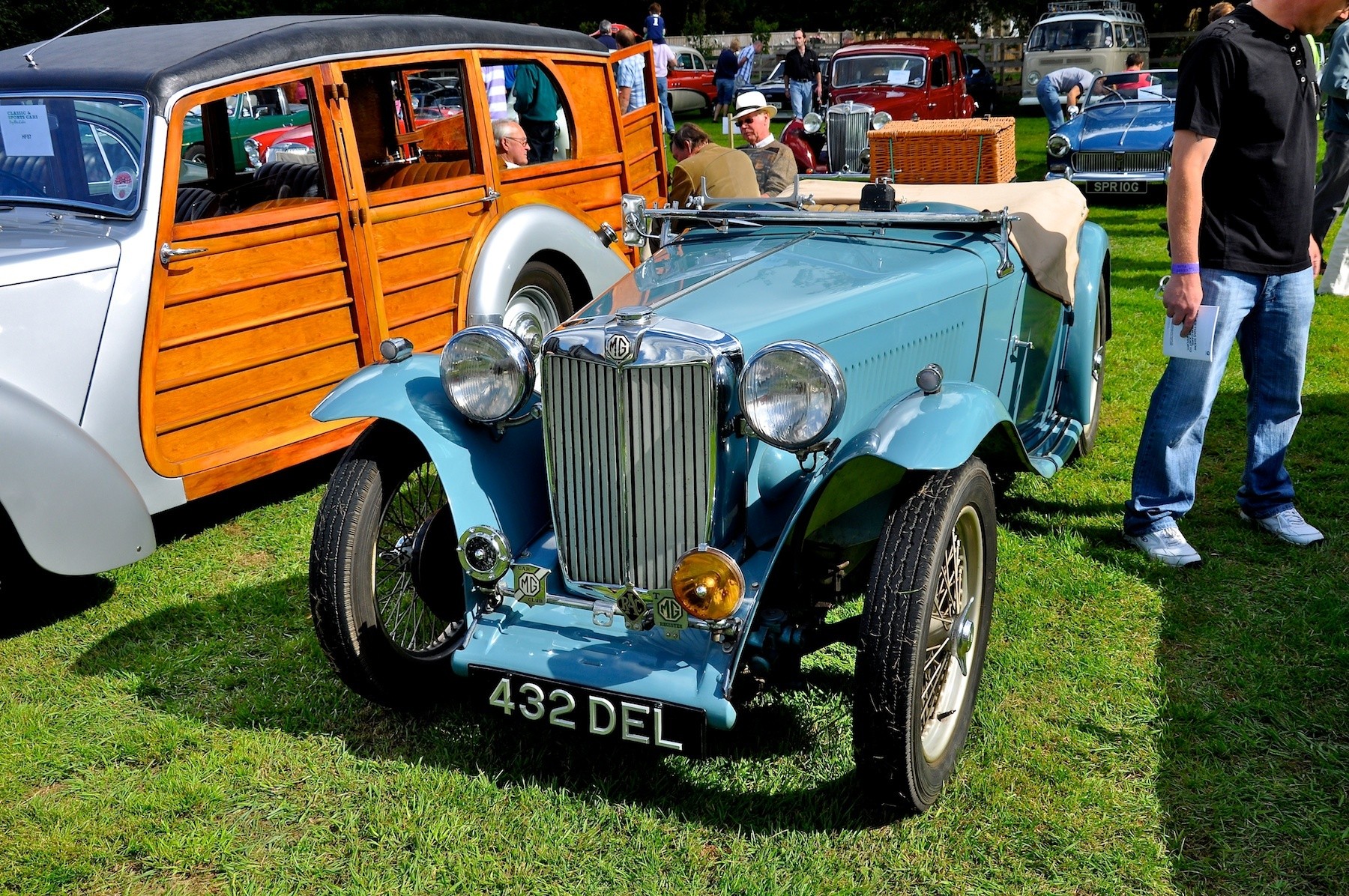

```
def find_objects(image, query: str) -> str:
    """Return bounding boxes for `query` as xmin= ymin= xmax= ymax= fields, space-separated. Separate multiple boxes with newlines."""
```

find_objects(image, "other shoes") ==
xmin=712 ymin=120 xmax=719 ymax=123
xmin=669 ymin=129 xmax=676 ymax=136
xmin=1319 ymin=258 xmax=1328 ymax=274
xmin=1159 ymin=223 xmax=1168 ymax=233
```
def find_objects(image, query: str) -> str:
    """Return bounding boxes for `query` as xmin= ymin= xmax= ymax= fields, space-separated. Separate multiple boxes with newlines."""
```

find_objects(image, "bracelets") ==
xmin=1171 ymin=263 xmax=1199 ymax=275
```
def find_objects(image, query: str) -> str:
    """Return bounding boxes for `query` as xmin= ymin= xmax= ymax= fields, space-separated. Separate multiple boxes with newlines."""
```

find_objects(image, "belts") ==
xmin=791 ymin=77 xmax=810 ymax=82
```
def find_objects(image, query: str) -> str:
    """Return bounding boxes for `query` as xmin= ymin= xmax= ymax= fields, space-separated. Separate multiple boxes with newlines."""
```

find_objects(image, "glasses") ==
xmin=499 ymin=136 xmax=528 ymax=148
xmin=735 ymin=110 xmax=765 ymax=127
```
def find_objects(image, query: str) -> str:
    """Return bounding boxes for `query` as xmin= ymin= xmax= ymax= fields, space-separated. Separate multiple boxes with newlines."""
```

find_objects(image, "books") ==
xmin=1163 ymin=305 xmax=1219 ymax=361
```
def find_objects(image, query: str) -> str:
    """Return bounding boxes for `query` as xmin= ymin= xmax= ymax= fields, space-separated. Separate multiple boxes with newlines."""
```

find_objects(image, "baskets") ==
xmin=868 ymin=116 xmax=1018 ymax=184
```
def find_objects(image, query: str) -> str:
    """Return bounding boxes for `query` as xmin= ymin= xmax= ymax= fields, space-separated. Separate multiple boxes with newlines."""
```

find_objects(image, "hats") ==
xmin=728 ymin=91 xmax=778 ymax=121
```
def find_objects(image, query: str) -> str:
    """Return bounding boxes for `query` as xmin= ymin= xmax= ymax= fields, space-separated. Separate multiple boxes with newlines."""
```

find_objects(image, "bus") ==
xmin=1018 ymin=0 xmax=1151 ymax=117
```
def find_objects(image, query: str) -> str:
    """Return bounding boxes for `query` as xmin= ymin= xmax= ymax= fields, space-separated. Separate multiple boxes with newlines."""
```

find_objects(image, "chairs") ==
xmin=368 ymin=158 xmax=472 ymax=191
xmin=176 ymin=187 xmax=221 ymax=223
xmin=249 ymin=163 xmax=321 ymax=200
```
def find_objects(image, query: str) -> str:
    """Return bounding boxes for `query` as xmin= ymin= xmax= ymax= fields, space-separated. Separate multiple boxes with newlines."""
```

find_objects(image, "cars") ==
xmin=732 ymin=53 xmax=831 ymax=122
xmin=1315 ymin=42 xmax=1330 ymax=120
xmin=667 ymin=44 xmax=719 ymax=117
xmin=0 ymin=12 xmax=669 ymax=629
xmin=307 ymin=164 xmax=1113 ymax=817
xmin=1041 ymin=68 xmax=1180 ymax=202
xmin=774 ymin=36 xmax=998 ymax=182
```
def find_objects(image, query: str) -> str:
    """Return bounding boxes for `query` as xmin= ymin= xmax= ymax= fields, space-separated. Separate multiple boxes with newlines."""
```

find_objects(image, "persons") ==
xmin=783 ymin=30 xmax=856 ymax=119
xmin=1124 ymin=0 xmax=1349 ymax=565
xmin=591 ymin=4 xmax=677 ymax=139
xmin=1037 ymin=67 xmax=1111 ymax=133
xmin=730 ymin=92 xmax=798 ymax=197
xmin=491 ymin=120 xmax=530 ymax=170
xmin=649 ymin=123 xmax=760 ymax=254
xmin=712 ymin=39 xmax=763 ymax=123
xmin=1113 ymin=54 xmax=1150 ymax=91
xmin=1311 ymin=21 xmax=1349 ymax=275
xmin=481 ymin=25 xmax=558 ymax=164
xmin=1209 ymin=2 xmax=1234 ymax=24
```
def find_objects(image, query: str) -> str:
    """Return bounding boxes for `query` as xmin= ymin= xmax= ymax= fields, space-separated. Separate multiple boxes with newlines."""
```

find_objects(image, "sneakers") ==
xmin=1121 ymin=525 xmax=1202 ymax=570
xmin=1239 ymin=507 xmax=1324 ymax=549
